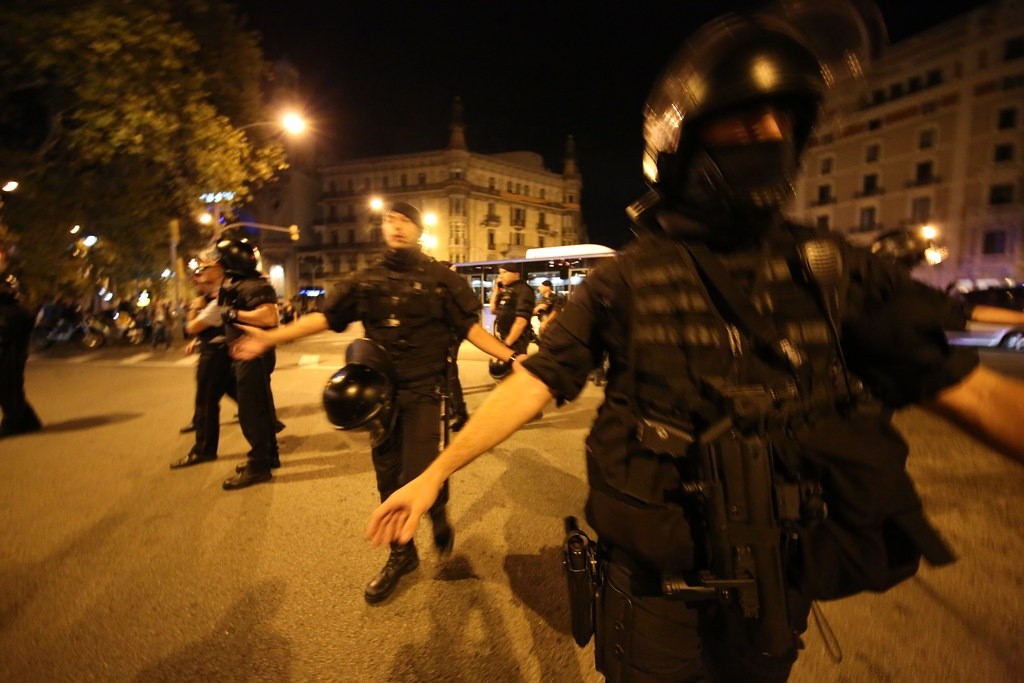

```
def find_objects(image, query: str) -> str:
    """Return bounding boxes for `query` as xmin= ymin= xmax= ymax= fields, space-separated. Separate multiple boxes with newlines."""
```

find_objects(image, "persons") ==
xmin=282 ymin=298 xmax=297 ymax=325
xmin=364 ymin=21 xmax=1024 ymax=683
xmin=227 ymin=203 xmax=530 ymax=605
xmin=149 ymin=296 xmax=172 ymax=350
xmin=167 ymin=228 xmax=286 ymax=490
xmin=531 ymin=280 xmax=560 ymax=339
xmin=0 ymin=251 xmax=44 ymax=439
xmin=488 ymin=261 xmax=543 ymax=424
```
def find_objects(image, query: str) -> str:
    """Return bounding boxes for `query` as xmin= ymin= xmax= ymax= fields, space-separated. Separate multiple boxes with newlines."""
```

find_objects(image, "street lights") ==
xmin=237 ymin=111 xmax=314 ymax=133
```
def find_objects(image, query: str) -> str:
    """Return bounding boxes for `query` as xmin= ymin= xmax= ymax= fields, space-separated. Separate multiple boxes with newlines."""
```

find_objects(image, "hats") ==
xmin=391 ymin=201 xmax=422 ymax=230
xmin=499 ymin=262 xmax=521 ymax=278
xmin=542 ymin=280 xmax=552 ymax=286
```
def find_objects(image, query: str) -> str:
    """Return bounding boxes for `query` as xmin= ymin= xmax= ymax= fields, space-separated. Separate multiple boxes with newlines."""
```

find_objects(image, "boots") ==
xmin=430 ymin=508 xmax=454 ymax=552
xmin=362 ymin=539 xmax=420 ymax=603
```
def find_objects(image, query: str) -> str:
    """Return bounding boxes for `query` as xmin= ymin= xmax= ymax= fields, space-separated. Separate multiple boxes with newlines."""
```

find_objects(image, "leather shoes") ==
xmin=170 ymin=453 xmax=218 ymax=469
xmin=179 ymin=421 xmax=195 ymax=433
xmin=222 ymin=460 xmax=280 ymax=490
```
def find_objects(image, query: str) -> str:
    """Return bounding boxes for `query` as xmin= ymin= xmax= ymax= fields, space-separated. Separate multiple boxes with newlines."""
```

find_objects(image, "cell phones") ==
xmin=498 ymin=282 xmax=503 ymax=288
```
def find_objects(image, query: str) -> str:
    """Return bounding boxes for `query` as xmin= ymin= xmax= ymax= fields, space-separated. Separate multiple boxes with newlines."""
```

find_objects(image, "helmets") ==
xmin=322 ymin=338 xmax=400 ymax=454
xmin=215 ymin=240 xmax=257 ymax=273
xmin=640 ymin=13 xmax=826 ymax=198
xmin=489 ymin=357 xmax=511 ymax=383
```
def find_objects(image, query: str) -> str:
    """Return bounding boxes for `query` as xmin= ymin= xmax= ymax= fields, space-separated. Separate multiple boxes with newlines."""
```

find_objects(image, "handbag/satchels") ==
xmin=773 ymin=402 xmax=959 ymax=600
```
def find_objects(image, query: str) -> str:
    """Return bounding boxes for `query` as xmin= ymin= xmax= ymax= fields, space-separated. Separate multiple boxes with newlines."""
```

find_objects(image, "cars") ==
xmin=948 ymin=279 xmax=1024 ymax=352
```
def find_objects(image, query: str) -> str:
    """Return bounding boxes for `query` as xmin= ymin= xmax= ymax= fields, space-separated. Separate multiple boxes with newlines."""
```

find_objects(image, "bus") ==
xmin=452 ymin=245 xmax=620 ymax=335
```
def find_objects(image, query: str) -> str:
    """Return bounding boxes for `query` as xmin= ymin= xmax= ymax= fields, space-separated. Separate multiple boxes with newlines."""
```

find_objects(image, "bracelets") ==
xmin=507 ymin=351 xmax=524 ymax=370
xmin=228 ymin=309 xmax=236 ymax=322
